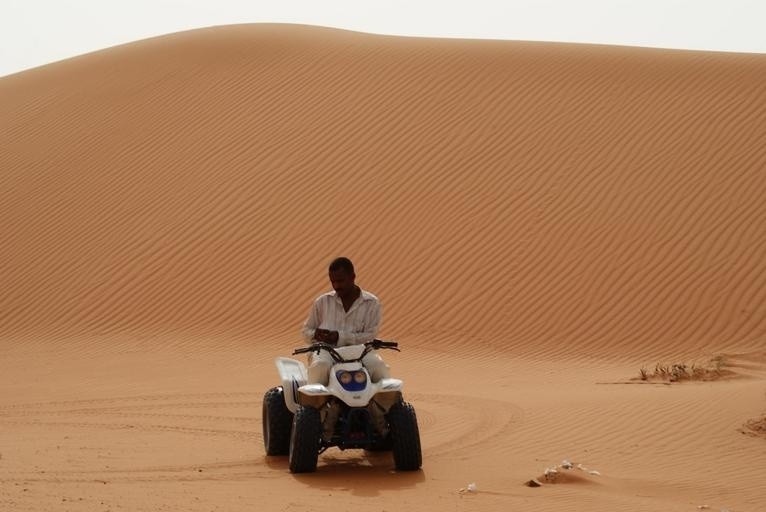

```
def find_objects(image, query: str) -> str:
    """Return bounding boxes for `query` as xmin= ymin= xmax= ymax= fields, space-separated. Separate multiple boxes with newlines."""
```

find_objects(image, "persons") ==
xmin=302 ymin=257 xmax=391 ymax=387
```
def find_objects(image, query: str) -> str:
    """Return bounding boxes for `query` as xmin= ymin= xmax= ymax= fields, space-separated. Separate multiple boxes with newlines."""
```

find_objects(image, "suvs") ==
xmin=261 ymin=337 xmax=426 ymax=482
